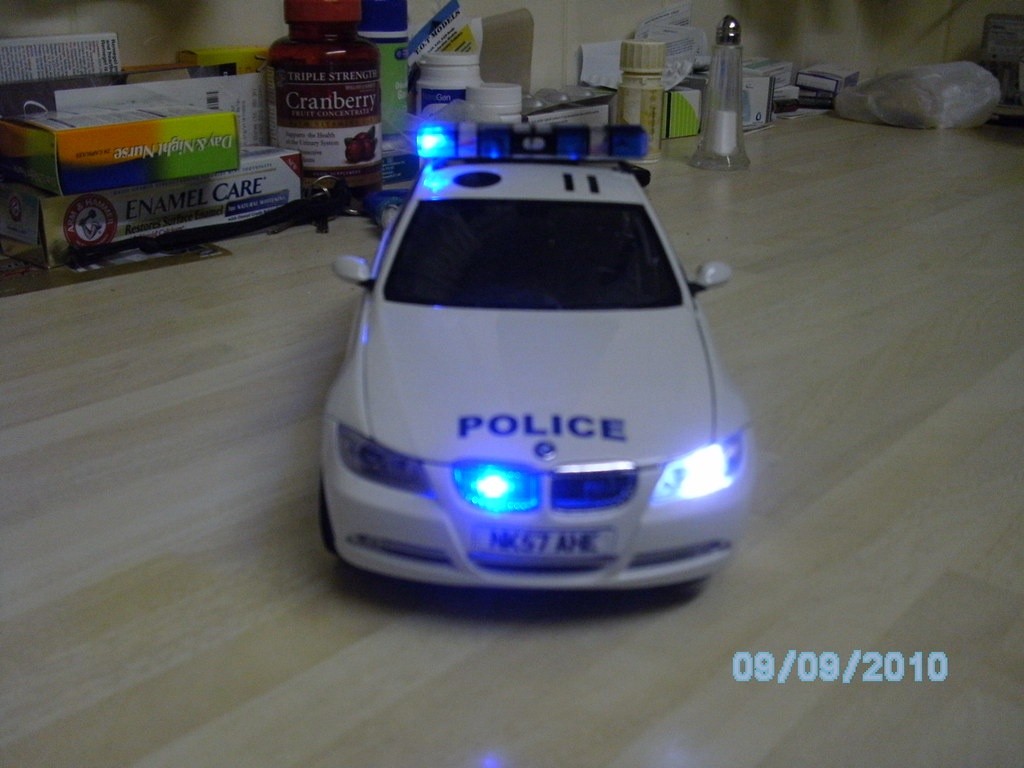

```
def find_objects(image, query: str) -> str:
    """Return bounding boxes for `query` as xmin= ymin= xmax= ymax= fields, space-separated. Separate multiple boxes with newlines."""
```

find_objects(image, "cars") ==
xmin=317 ymin=119 xmax=756 ymax=605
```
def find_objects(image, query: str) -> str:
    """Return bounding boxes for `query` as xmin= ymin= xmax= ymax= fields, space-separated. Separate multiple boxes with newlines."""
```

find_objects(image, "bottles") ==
xmin=265 ymin=0 xmax=385 ymax=214
xmin=465 ymin=83 xmax=522 ymax=127
xmin=690 ymin=14 xmax=751 ymax=171
xmin=416 ymin=51 xmax=483 ymax=117
xmin=616 ymin=39 xmax=667 ymax=162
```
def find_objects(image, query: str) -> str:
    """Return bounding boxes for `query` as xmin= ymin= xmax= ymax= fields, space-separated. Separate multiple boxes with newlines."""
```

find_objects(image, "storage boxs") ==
xmin=521 ymin=55 xmax=859 ymax=140
xmin=0 ymin=31 xmax=302 ymax=269
